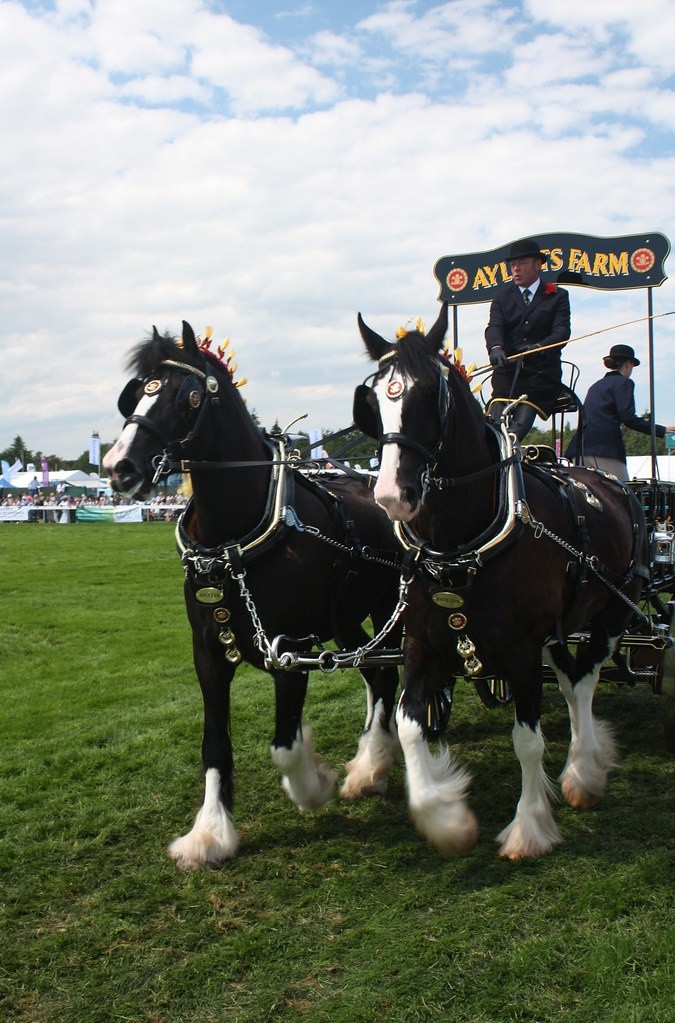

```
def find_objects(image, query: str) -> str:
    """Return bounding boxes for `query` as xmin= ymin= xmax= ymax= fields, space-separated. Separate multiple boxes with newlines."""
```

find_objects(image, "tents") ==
xmin=0 ymin=466 xmax=98 ymax=498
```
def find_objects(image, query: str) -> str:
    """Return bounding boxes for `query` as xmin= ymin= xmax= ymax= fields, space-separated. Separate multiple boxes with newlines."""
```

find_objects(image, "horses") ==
xmin=357 ymin=302 xmax=650 ymax=861
xmin=101 ymin=321 xmax=409 ymax=873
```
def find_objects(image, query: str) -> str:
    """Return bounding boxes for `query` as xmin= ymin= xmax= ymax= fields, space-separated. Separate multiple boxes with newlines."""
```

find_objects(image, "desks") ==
xmin=0 ymin=504 xmax=188 ymax=521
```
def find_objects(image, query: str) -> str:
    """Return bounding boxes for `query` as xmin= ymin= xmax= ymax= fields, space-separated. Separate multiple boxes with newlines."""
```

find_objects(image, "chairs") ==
xmin=482 ymin=360 xmax=598 ymax=466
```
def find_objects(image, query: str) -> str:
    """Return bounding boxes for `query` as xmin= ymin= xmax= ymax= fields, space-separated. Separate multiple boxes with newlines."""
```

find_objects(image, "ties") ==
xmin=523 ymin=289 xmax=532 ymax=306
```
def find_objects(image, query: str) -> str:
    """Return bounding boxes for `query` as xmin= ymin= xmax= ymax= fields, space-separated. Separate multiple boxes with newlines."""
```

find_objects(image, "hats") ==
xmin=504 ymin=239 xmax=547 ymax=265
xmin=7 ymin=494 xmax=12 ymax=498
xmin=603 ymin=345 xmax=640 ymax=367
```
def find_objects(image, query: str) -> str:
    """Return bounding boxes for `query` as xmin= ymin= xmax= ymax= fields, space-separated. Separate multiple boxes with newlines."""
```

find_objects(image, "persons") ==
xmin=484 ymin=239 xmax=571 ymax=445
xmin=565 ymin=344 xmax=675 ymax=484
xmin=0 ymin=492 xmax=189 ymax=523
xmin=56 ymin=481 xmax=65 ymax=493
xmin=27 ymin=476 xmax=40 ymax=495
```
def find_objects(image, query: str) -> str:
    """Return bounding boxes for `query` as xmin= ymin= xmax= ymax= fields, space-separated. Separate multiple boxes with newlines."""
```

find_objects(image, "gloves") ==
xmin=517 ymin=344 xmax=543 ymax=365
xmin=490 ymin=347 xmax=508 ymax=371
